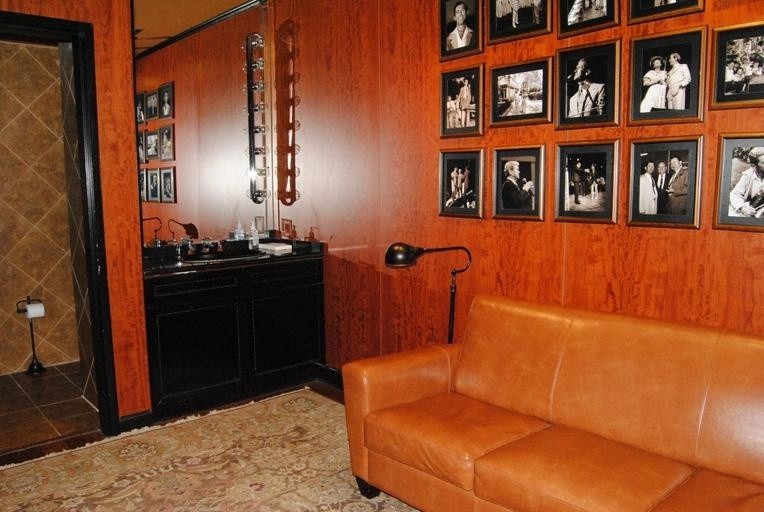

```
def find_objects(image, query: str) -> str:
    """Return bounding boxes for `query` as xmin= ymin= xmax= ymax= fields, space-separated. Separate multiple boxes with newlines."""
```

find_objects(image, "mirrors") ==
xmin=130 ymin=1 xmax=281 ymax=248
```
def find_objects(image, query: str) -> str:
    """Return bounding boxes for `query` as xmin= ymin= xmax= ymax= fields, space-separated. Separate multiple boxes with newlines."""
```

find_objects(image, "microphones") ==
xmin=522 ymin=177 xmax=535 ymax=197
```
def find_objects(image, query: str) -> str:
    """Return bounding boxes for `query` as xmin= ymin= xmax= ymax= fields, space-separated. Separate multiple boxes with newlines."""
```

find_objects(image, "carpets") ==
xmin=0 ymin=385 xmax=425 ymax=512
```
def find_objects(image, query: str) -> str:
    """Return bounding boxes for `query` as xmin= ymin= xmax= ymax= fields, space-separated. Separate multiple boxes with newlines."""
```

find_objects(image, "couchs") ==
xmin=341 ymin=291 xmax=764 ymax=512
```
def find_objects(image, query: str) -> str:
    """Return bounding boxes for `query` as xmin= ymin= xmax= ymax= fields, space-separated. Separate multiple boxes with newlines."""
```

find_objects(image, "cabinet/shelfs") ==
xmin=143 ymin=257 xmax=326 ymax=412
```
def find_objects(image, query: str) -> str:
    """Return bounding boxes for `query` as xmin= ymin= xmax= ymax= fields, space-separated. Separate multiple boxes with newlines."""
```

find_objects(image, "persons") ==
xmin=568 ymin=58 xmax=607 ymax=118
xmin=639 ymin=161 xmax=657 ymax=214
xmin=665 ymin=156 xmax=688 ymax=215
xmin=150 ymin=175 xmax=156 ymax=198
xmin=161 ymin=131 xmax=169 ymax=158
xmin=502 ymin=161 xmax=533 ymax=209
xmin=164 ymin=176 xmax=171 ymax=198
xmin=148 ymin=97 xmax=156 ymax=118
xmin=666 ymin=53 xmax=691 ymax=110
xmin=450 ymin=166 xmax=457 ymax=199
xmin=136 ymin=103 xmax=143 ymax=122
xmin=587 ymin=164 xmax=603 ymax=199
xmin=514 ymin=91 xmax=526 ymax=114
xmin=729 ymin=147 xmax=763 ymax=217
xmin=457 ymin=169 xmax=463 ymax=197
xmin=725 ymin=53 xmax=764 ymax=94
xmin=567 ymin=1 xmax=600 ymax=26
xmin=446 ymin=80 xmax=471 ymax=130
xmin=463 ymin=167 xmax=472 ymax=190
xmin=496 ymin=0 xmax=541 ymax=28
xmin=446 ymin=2 xmax=473 ymax=51
xmin=162 ymin=92 xmax=170 ymax=116
xmin=573 ymin=162 xmax=589 ymax=204
xmin=639 ymin=57 xmax=667 ymax=113
xmin=654 ymin=162 xmax=671 ymax=214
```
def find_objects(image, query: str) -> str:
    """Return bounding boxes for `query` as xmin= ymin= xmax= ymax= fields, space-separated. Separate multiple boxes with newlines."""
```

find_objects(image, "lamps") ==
xmin=164 ymin=219 xmax=201 ymax=240
xmin=384 ymin=242 xmax=474 ymax=341
xmin=246 ymin=31 xmax=271 ymax=203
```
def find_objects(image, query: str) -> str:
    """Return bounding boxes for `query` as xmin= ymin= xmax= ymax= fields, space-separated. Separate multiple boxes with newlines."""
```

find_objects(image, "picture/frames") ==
xmin=135 ymin=79 xmax=177 ymax=201
